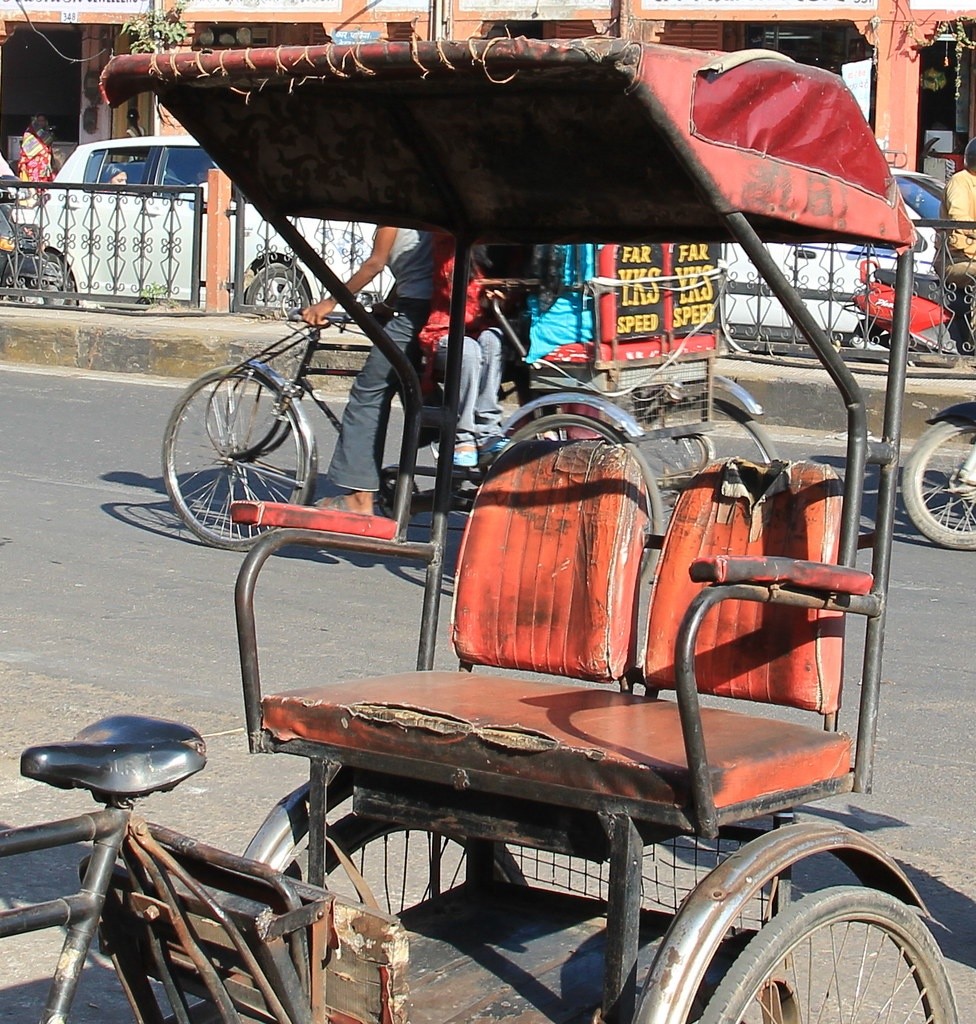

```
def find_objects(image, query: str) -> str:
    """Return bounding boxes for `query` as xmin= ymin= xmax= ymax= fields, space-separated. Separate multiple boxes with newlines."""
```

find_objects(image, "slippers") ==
xmin=313 ymin=495 xmax=351 ymax=513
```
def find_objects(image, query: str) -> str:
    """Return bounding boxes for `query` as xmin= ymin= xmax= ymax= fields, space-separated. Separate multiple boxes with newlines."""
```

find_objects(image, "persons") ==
xmin=933 ymin=137 xmax=976 ymax=356
xmin=419 ymin=232 xmax=510 ymax=467
xmin=302 ymin=225 xmax=434 ymax=517
xmin=99 ymin=161 xmax=127 ymax=184
xmin=16 ymin=113 xmax=58 ymax=206
xmin=121 ymin=108 xmax=146 ymax=161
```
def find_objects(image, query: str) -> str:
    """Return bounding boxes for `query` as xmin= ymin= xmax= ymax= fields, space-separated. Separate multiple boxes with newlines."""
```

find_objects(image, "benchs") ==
xmin=227 ymin=440 xmax=878 ymax=842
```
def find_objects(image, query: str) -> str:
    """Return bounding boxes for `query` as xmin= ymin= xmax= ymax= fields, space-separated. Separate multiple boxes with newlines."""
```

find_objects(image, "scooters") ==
xmin=848 ymin=258 xmax=976 ymax=360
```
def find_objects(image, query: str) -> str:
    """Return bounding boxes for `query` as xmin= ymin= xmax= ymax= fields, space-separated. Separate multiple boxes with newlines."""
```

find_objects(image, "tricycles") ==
xmin=0 ymin=35 xmax=962 ymax=1024
xmin=160 ymin=234 xmax=784 ymax=605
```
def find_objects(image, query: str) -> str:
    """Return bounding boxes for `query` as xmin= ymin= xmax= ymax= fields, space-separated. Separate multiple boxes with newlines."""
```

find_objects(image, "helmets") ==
xmin=963 ymin=137 xmax=976 ymax=173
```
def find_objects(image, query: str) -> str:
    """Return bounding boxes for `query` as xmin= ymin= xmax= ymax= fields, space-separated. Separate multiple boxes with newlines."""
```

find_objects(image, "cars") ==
xmin=29 ymin=134 xmax=405 ymax=322
xmin=717 ymin=166 xmax=955 ymax=349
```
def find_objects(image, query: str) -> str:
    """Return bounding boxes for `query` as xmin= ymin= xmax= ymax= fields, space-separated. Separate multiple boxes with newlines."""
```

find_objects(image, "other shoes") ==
xmin=452 ymin=442 xmax=477 ymax=467
xmin=478 ymin=435 xmax=511 ymax=455
